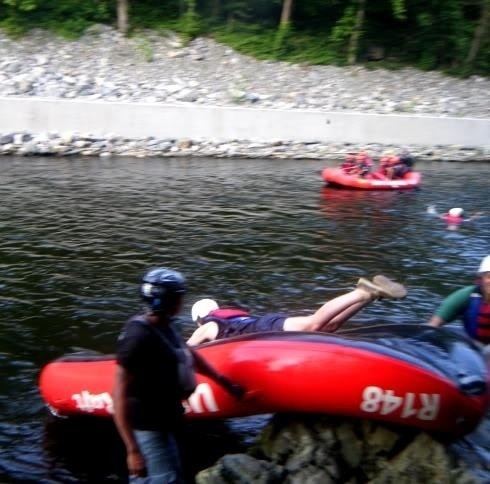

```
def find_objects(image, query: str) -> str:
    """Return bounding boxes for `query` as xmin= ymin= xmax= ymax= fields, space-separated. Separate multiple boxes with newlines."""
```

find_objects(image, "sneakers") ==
xmin=357 ymin=274 xmax=407 ymax=301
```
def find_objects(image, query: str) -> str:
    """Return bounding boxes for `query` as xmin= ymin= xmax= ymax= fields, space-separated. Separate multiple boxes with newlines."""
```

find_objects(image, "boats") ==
xmin=324 ymin=168 xmax=422 ymax=190
xmin=39 ymin=320 xmax=489 ymax=431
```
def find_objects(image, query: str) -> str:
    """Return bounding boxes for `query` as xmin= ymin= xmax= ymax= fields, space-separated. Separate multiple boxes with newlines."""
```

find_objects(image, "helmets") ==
xmin=191 ymin=298 xmax=220 ymax=322
xmin=477 ymin=256 xmax=490 ymax=272
xmin=141 ymin=268 xmax=189 ymax=298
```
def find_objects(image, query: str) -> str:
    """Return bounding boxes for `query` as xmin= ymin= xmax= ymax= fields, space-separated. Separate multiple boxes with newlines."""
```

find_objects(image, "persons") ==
xmin=341 ymin=147 xmax=415 ymax=181
xmin=112 ymin=267 xmax=197 ymax=484
xmin=185 ymin=275 xmax=408 ymax=346
xmin=440 ymin=207 xmax=472 ymax=224
xmin=425 ymin=255 xmax=490 ymax=348
